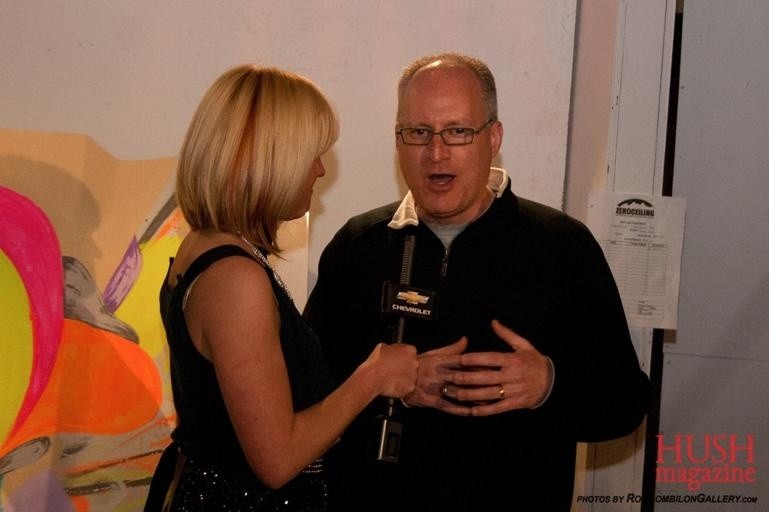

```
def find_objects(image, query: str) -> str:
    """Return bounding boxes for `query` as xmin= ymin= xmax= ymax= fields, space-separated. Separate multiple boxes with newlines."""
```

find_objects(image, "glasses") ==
xmin=396 ymin=120 xmax=495 ymax=145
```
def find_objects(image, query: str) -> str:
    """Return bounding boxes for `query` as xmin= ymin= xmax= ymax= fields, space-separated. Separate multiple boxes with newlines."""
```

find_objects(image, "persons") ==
xmin=302 ymin=53 xmax=650 ymax=510
xmin=145 ymin=65 xmax=419 ymax=510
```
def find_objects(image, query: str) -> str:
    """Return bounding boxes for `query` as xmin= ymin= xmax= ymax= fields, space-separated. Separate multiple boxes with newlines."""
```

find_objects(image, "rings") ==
xmin=441 ymin=383 xmax=448 ymax=396
xmin=498 ymin=383 xmax=507 ymax=402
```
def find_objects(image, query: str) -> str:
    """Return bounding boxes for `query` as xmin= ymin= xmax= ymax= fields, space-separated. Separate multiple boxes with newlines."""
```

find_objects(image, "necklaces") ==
xmin=236 ymin=226 xmax=295 ymax=301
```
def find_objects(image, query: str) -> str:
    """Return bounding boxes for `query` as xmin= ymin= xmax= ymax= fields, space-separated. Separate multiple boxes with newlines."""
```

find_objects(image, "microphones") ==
xmin=366 ymin=231 xmax=437 ymax=468
